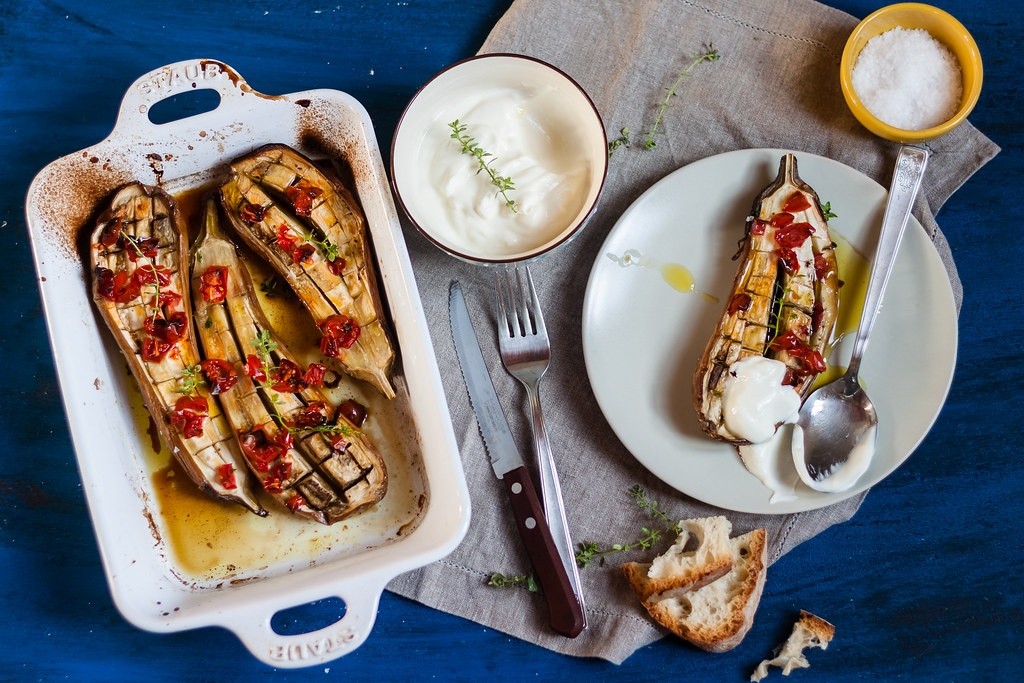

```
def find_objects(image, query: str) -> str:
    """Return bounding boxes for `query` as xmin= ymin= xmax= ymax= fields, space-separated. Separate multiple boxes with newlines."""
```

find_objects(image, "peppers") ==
xmin=731 ymin=188 xmax=826 ymax=380
xmin=101 ymin=184 xmax=359 ymax=511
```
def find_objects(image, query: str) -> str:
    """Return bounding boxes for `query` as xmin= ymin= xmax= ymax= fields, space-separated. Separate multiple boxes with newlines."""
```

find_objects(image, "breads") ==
xmin=624 ymin=516 xmax=768 ymax=652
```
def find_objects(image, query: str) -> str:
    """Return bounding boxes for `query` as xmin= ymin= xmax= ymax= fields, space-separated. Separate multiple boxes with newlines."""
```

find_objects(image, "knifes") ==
xmin=447 ymin=281 xmax=584 ymax=640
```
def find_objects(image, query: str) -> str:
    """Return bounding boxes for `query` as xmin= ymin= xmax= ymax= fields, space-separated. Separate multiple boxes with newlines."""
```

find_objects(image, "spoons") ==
xmin=790 ymin=144 xmax=929 ymax=493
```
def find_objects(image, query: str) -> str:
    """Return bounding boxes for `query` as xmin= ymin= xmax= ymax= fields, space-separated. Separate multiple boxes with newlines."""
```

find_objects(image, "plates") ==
xmin=22 ymin=57 xmax=472 ymax=668
xmin=578 ymin=147 xmax=959 ymax=516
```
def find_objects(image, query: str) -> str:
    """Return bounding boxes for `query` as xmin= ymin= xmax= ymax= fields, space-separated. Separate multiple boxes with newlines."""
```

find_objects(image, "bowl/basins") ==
xmin=388 ymin=54 xmax=608 ymax=268
xmin=839 ymin=3 xmax=984 ymax=145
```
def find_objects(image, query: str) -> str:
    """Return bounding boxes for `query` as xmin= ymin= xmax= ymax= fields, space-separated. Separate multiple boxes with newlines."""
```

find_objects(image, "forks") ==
xmin=492 ymin=264 xmax=587 ymax=633
